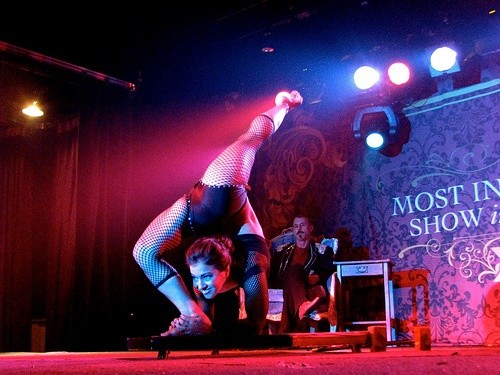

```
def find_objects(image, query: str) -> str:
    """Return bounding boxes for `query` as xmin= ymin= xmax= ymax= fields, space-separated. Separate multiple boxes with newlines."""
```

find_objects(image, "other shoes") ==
xmin=299 ymin=297 xmax=321 ymax=320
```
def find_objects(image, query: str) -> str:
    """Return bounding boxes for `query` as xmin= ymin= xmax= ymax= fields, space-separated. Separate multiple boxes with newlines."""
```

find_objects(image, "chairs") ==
xmin=260 ymin=231 xmax=338 ymax=351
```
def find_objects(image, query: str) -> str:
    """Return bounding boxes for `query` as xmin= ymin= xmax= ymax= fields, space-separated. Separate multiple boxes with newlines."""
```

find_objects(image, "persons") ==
xmin=132 ymin=90 xmax=303 ymax=336
xmin=268 ymin=214 xmax=333 ymax=333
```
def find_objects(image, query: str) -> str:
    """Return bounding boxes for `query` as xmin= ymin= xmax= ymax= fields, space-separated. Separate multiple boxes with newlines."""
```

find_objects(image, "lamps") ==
xmin=424 ymin=41 xmax=461 ymax=95
xmin=22 ymin=101 xmax=44 ymax=118
xmin=352 ymin=105 xmax=401 ymax=151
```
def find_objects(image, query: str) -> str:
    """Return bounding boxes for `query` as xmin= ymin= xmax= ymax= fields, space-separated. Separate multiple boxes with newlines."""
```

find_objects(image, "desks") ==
xmin=333 ymin=259 xmax=397 ymax=347
